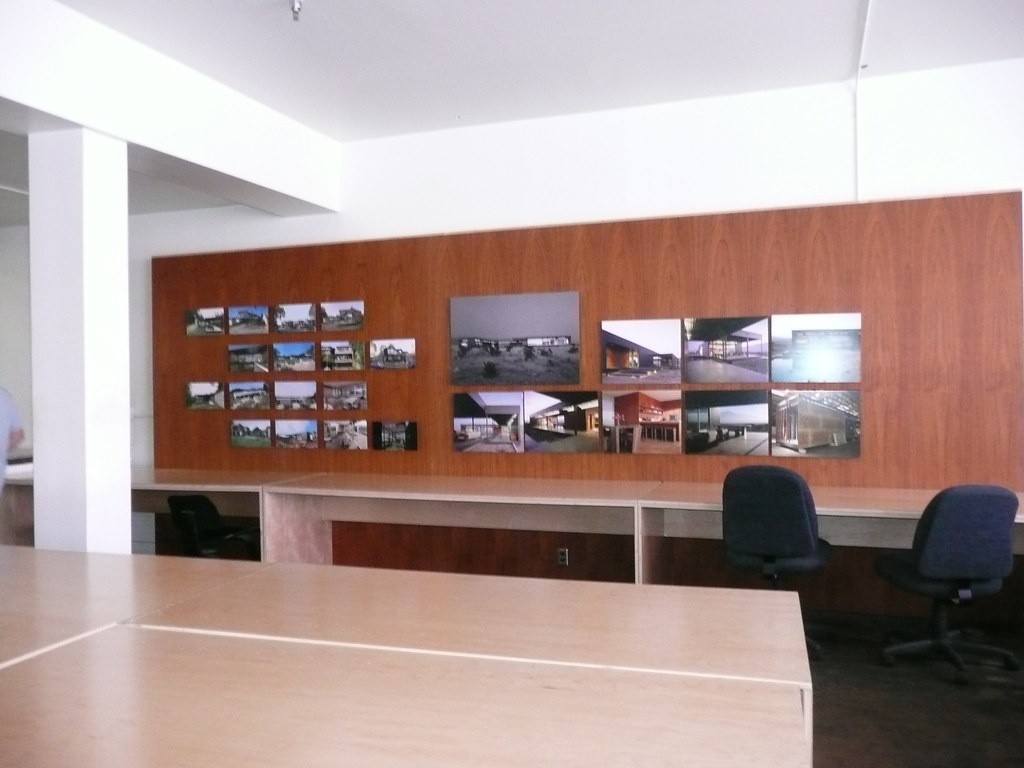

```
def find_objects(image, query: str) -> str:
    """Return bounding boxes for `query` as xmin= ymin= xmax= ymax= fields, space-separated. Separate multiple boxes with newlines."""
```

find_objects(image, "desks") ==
xmin=130 ymin=464 xmax=1024 ymax=587
xmin=0 ymin=545 xmax=814 ymax=768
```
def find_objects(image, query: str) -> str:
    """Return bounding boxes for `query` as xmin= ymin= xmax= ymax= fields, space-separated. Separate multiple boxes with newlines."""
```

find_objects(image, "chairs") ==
xmin=715 ymin=464 xmax=834 ymax=657
xmin=168 ymin=495 xmax=260 ymax=559
xmin=873 ymin=485 xmax=1021 ymax=686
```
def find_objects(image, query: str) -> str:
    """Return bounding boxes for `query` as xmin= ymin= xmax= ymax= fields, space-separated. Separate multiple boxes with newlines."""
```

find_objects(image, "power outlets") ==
xmin=558 ymin=547 xmax=568 ymax=566
